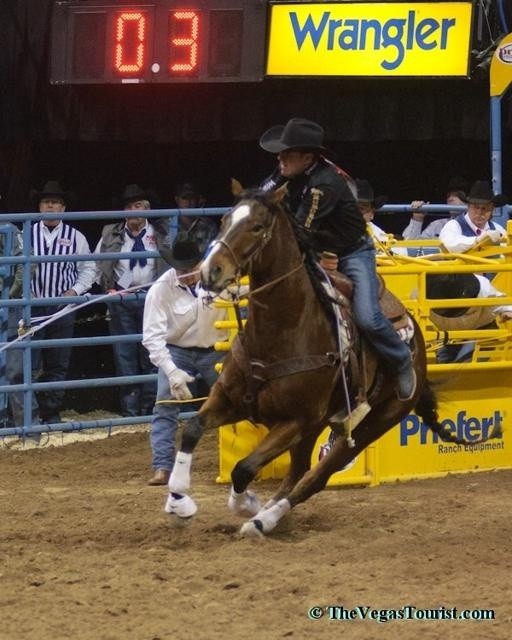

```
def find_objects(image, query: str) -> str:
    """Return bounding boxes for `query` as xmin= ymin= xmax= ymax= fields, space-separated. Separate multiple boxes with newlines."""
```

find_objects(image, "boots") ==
xmin=120 ymin=391 xmax=157 ymax=417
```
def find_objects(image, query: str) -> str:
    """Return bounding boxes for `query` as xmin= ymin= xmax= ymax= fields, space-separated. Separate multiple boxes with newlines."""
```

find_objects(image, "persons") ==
xmin=258 ymin=117 xmax=418 ymax=402
xmin=142 ymin=232 xmax=233 ymax=486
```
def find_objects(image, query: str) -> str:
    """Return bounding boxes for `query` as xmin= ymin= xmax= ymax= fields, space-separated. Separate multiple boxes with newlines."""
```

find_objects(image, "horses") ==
xmin=164 ymin=179 xmax=502 ymax=540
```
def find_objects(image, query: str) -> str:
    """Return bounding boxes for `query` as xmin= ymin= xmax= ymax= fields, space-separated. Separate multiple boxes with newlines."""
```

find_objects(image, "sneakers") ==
xmin=391 ymin=346 xmax=417 ymax=401
xmin=0 ymin=409 xmax=68 ymax=437
xmin=147 ymin=469 xmax=171 ymax=484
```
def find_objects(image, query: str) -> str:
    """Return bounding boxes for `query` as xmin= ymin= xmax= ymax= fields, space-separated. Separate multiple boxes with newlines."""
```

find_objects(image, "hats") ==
xmin=112 ymin=184 xmax=162 ymax=206
xmin=174 ymin=181 xmax=200 ymax=198
xmin=30 ymin=179 xmax=80 ymax=198
xmin=426 ymin=257 xmax=480 ymax=317
xmin=443 ymin=175 xmax=510 ymax=207
xmin=259 ymin=116 xmax=329 ymax=154
xmin=352 ymin=176 xmax=390 ymax=210
xmin=156 ymin=230 xmax=205 ymax=271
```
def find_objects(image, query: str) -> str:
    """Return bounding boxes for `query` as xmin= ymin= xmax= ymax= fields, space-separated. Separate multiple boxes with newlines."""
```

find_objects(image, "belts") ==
xmin=167 ymin=339 xmax=229 ymax=353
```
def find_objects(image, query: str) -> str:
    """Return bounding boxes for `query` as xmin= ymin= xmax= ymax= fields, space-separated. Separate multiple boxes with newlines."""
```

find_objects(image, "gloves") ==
xmin=169 ymin=369 xmax=196 ymax=401
